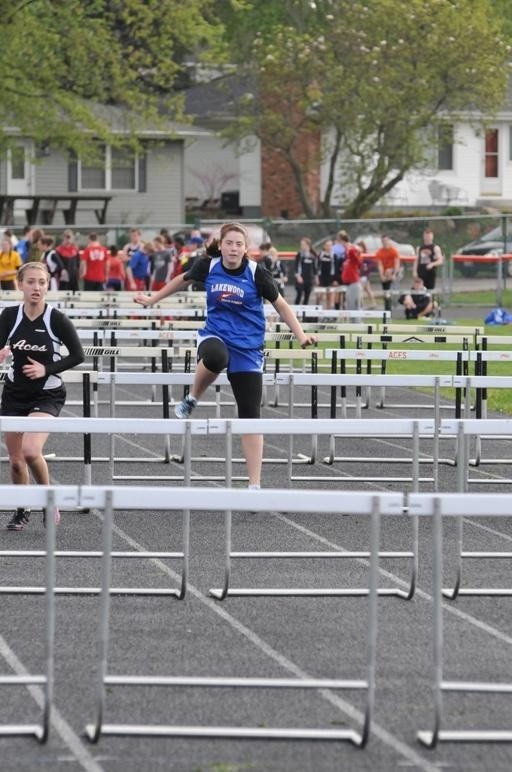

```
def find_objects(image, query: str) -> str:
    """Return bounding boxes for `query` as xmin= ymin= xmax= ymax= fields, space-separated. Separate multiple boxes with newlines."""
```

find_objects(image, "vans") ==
xmin=170 ymin=224 xmax=281 ymax=262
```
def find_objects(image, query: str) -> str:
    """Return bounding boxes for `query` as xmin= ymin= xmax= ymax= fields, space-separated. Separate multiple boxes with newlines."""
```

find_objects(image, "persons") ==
xmin=412 ymin=230 xmax=442 ymax=289
xmin=399 ymin=277 xmax=439 ymax=320
xmin=376 ymin=235 xmax=401 ymax=310
xmin=332 ymin=241 xmax=346 ymax=310
xmin=356 ymin=240 xmax=377 ymax=309
xmin=336 ymin=230 xmax=364 ymax=310
xmin=317 ymin=238 xmax=338 ymax=311
xmin=134 ymin=222 xmax=317 ymax=490
xmin=0 ymin=261 xmax=86 ymax=532
xmin=1 ymin=226 xmax=289 ymax=320
xmin=293 ymin=237 xmax=320 ymax=305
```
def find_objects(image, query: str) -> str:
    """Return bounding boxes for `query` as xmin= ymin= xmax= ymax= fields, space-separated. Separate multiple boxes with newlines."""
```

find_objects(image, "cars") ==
xmin=303 ymin=227 xmax=416 ymax=285
xmin=450 ymin=221 xmax=512 ymax=279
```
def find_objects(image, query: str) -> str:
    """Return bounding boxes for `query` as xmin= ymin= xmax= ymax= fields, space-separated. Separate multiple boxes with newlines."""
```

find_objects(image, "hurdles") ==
xmin=0 ymin=485 xmax=79 ymax=747
xmin=406 ymin=490 xmax=512 ymax=750
xmin=0 ymin=288 xmax=512 ymax=601
xmin=75 ymin=480 xmax=405 ymax=749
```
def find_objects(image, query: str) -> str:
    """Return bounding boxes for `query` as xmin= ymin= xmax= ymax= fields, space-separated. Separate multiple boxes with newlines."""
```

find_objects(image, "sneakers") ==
xmin=175 ymin=394 xmax=196 ymax=418
xmin=43 ymin=506 xmax=61 ymax=528
xmin=7 ymin=507 xmax=31 ymax=530
